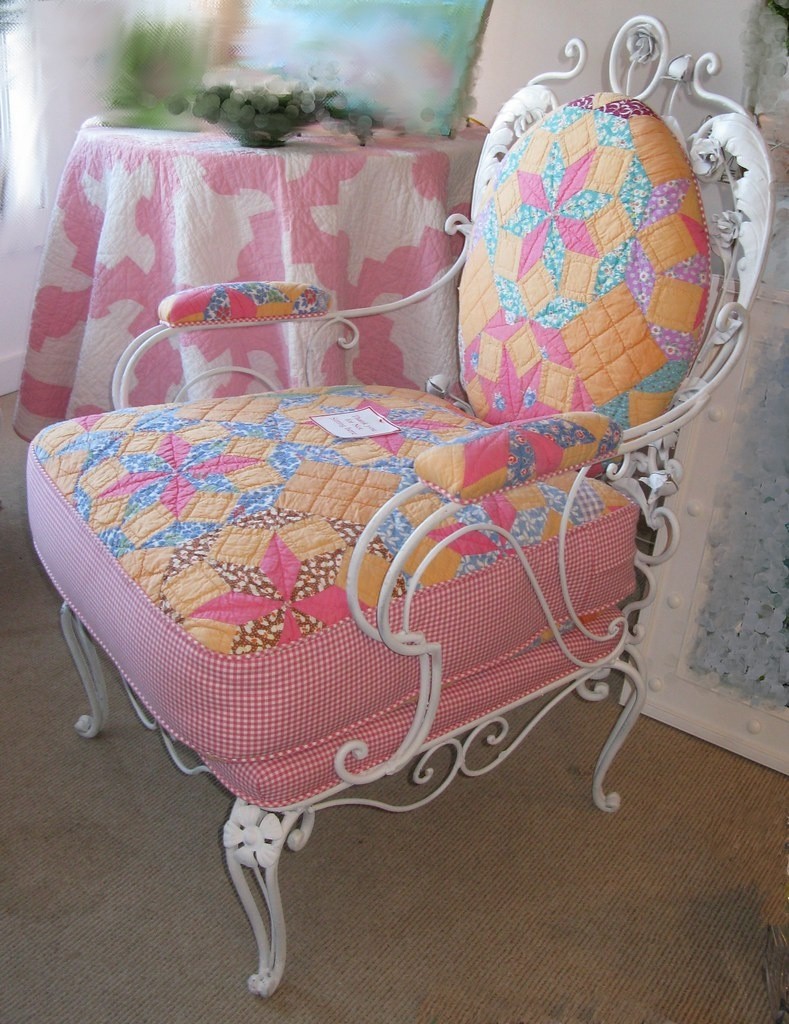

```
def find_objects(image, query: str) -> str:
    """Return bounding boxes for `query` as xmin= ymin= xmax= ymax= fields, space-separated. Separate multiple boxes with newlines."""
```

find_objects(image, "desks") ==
xmin=10 ymin=112 xmax=493 ymax=441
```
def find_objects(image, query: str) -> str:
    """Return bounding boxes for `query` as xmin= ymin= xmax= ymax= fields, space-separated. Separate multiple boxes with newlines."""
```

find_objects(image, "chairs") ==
xmin=23 ymin=14 xmax=776 ymax=1001
